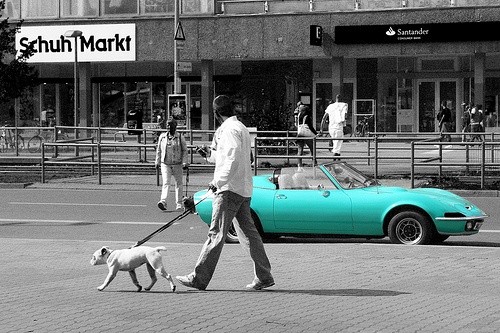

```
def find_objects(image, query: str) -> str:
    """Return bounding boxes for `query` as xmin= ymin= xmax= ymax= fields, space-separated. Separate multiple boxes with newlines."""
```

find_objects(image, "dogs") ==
xmin=88 ymin=245 xmax=177 ymax=293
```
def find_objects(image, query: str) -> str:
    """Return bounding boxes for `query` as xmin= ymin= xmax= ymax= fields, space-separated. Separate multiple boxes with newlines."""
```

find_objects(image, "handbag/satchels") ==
xmin=298 ymin=114 xmax=315 ymax=136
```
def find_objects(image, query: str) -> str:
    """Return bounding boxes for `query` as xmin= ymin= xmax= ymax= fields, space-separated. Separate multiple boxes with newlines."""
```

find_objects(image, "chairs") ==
xmin=278 ymin=173 xmax=310 ymax=189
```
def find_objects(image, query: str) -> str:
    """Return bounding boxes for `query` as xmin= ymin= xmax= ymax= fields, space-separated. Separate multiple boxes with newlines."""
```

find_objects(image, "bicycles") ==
xmin=0 ymin=117 xmax=70 ymax=154
xmin=353 ymin=116 xmax=386 ymax=143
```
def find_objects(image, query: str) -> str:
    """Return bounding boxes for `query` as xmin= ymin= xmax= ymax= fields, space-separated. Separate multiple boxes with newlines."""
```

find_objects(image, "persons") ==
xmin=438 ymin=100 xmax=484 ymax=147
xmin=321 ymin=94 xmax=348 ymax=161
xmin=175 ymin=94 xmax=275 ymax=290
xmin=294 ymin=101 xmax=321 ymax=167
xmin=154 ymin=118 xmax=190 ymax=210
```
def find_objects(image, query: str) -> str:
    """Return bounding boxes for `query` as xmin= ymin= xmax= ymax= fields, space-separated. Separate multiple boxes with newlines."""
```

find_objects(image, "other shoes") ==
xmin=246 ymin=280 xmax=275 ymax=289
xmin=176 ymin=273 xmax=206 ymax=290
xmin=176 ymin=205 xmax=183 ymax=210
xmin=297 ymin=167 xmax=305 ymax=172
xmin=157 ymin=200 xmax=167 ymax=210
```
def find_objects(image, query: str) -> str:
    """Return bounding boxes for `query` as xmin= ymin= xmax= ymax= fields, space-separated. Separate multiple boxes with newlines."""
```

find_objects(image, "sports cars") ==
xmin=193 ymin=162 xmax=489 ymax=246
